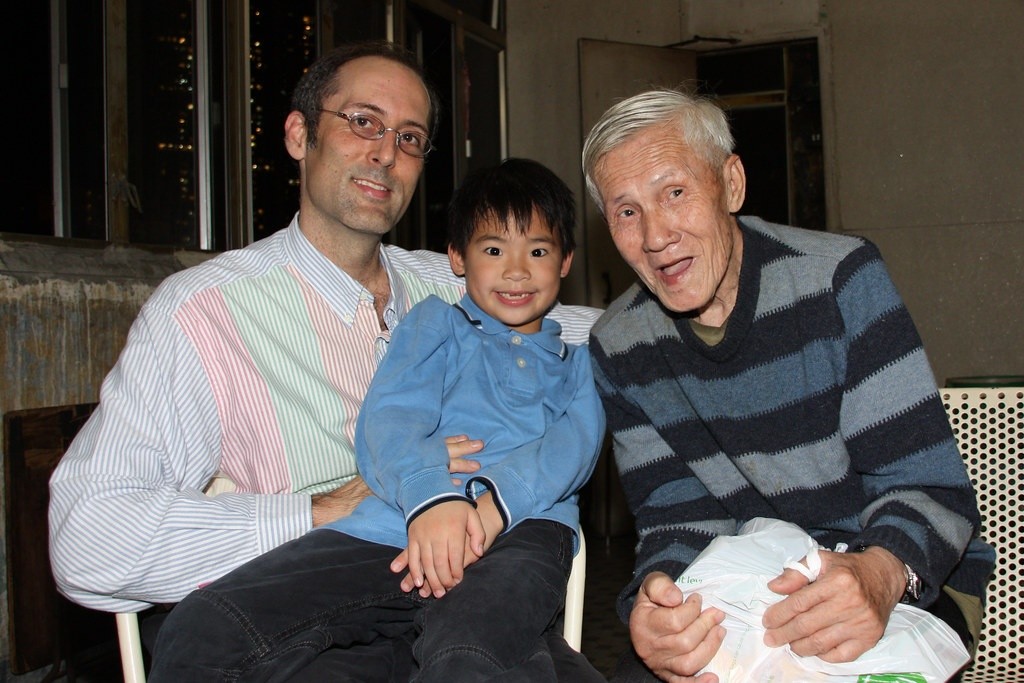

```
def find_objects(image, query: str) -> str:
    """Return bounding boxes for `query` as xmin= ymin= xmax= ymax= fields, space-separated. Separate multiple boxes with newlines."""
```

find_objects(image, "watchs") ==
xmin=904 ymin=562 xmax=923 ymax=599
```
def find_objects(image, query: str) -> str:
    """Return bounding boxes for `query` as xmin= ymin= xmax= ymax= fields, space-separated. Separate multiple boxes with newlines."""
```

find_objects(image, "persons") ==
xmin=582 ymin=90 xmax=996 ymax=683
xmin=149 ymin=158 xmax=607 ymax=683
xmin=49 ymin=47 xmax=605 ymax=683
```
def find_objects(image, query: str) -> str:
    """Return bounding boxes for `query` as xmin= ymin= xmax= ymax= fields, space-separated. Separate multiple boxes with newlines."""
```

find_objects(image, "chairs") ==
xmin=115 ymin=526 xmax=587 ymax=683
xmin=936 ymin=386 xmax=1024 ymax=683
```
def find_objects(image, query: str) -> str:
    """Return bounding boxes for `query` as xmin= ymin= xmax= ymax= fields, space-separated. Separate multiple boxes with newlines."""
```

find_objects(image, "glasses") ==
xmin=318 ymin=108 xmax=437 ymax=159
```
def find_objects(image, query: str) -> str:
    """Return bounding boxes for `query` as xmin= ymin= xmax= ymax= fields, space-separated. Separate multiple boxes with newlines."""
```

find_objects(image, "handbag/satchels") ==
xmin=674 ymin=517 xmax=971 ymax=683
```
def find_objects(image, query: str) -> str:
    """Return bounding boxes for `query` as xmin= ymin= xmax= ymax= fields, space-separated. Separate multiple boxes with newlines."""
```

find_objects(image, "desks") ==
xmin=1 ymin=401 xmax=169 ymax=683
